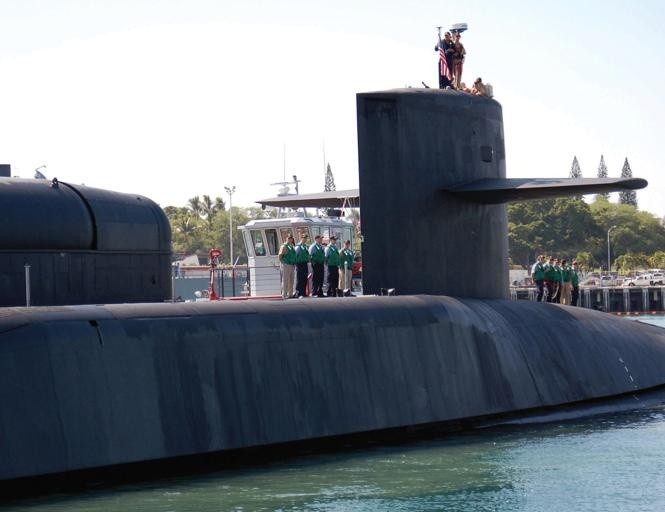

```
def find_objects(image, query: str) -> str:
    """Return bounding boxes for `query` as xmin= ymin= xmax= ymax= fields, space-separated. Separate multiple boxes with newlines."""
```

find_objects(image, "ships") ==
xmin=0 ymin=87 xmax=665 ymax=484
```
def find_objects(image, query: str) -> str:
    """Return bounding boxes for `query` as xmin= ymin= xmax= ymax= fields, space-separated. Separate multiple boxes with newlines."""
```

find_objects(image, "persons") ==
xmin=278 ymin=232 xmax=358 ymax=299
xmin=434 ymin=32 xmax=456 ymax=90
xmin=530 ymin=254 xmax=580 ymax=306
xmin=452 ymin=34 xmax=466 ymax=90
xmin=464 ymin=77 xmax=487 ymax=96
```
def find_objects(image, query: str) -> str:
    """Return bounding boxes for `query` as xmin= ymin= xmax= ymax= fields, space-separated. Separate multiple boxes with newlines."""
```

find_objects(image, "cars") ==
xmin=512 ymin=268 xmax=665 ymax=287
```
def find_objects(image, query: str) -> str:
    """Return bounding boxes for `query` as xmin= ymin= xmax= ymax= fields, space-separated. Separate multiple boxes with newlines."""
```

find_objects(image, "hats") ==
xmin=329 ymin=236 xmax=338 ymax=241
xmin=301 ymin=233 xmax=308 ymax=238
xmin=314 ymin=234 xmax=324 ymax=240
xmin=287 ymin=234 xmax=294 ymax=240
xmin=344 ymin=239 xmax=350 ymax=245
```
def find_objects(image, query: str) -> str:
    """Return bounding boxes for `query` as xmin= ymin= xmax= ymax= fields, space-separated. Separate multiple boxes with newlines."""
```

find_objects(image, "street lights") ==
xmin=607 ymin=225 xmax=617 ymax=270
xmin=225 ymin=186 xmax=235 ymax=264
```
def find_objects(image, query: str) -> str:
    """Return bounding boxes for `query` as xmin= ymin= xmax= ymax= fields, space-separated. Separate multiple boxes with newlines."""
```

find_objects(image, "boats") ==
xmin=183 ymin=217 xmax=355 ymax=302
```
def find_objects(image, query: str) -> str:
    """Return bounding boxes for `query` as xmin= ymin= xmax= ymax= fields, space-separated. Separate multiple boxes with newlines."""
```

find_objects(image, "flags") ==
xmin=437 ymin=33 xmax=452 ymax=81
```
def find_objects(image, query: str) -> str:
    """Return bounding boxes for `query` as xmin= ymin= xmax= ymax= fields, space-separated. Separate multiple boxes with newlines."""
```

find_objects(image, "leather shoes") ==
xmin=282 ymin=293 xmax=356 ymax=300
